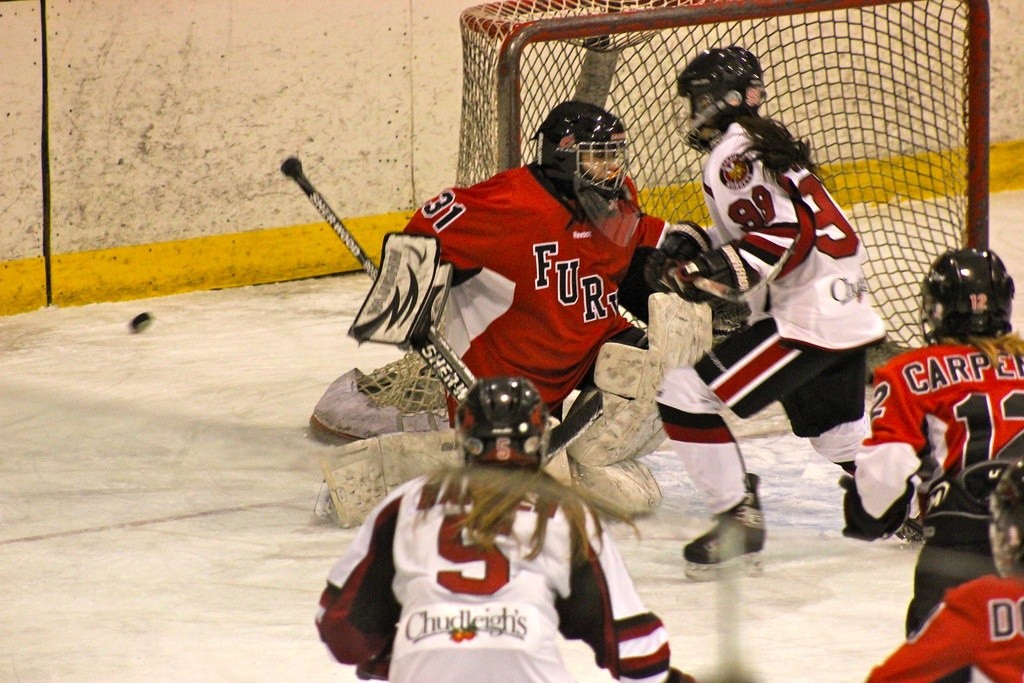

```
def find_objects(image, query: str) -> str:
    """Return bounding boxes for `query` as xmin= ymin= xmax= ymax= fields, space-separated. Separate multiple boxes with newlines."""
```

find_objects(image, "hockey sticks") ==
xmin=279 ymin=153 xmax=607 ymax=463
xmin=668 ymin=234 xmax=802 ymax=308
xmin=837 ymin=474 xmax=922 ymax=534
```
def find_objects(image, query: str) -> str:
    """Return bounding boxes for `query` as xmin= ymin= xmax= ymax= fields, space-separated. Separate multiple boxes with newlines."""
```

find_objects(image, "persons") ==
xmin=315 ymin=374 xmax=694 ymax=683
xmin=645 ymin=46 xmax=922 ymax=583
xmin=843 ymin=248 xmax=1024 ymax=683
xmin=865 ymin=459 xmax=1024 ymax=683
xmin=323 ymin=99 xmax=713 ymax=528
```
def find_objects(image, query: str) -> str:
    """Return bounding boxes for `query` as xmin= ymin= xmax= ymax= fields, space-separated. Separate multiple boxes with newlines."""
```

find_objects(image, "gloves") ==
xmin=661 ymin=221 xmax=713 ymax=262
xmin=665 ymin=239 xmax=760 ymax=307
xmin=840 ymin=473 xmax=915 ymax=542
xmin=639 ymin=246 xmax=671 ymax=293
xmin=668 ymin=668 xmax=695 ymax=683
xmin=356 ymin=628 xmax=397 ymax=681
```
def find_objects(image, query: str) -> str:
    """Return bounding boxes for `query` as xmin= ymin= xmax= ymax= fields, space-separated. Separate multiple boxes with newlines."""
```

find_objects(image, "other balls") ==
xmin=130 ymin=312 xmax=152 ymax=334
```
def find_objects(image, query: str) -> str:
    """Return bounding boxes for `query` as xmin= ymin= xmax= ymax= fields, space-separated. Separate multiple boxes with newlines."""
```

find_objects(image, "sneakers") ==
xmin=839 ymin=474 xmax=925 ymax=544
xmin=684 ymin=473 xmax=768 ymax=581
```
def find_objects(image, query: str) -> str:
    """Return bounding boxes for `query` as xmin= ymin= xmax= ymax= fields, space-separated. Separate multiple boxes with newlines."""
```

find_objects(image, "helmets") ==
xmin=993 ymin=458 xmax=1024 ymax=568
xmin=672 ymin=46 xmax=765 ymax=154
xmin=920 ymin=249 xmax=1015 ymax=347
xmin=454 ymin=376 xmax=546 ymax=472
xmin=542 ymin=100 xmax=628 ymax=198
